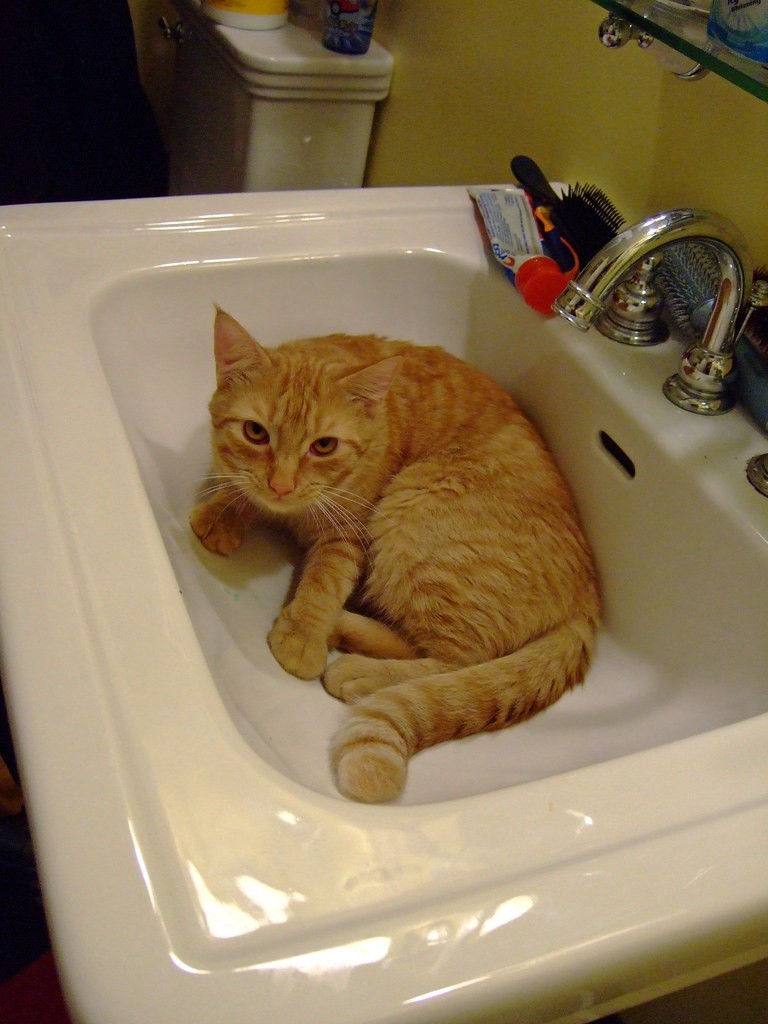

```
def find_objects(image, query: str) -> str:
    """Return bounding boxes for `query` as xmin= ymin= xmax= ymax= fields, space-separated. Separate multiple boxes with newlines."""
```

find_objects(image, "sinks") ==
xmin=2 ymin=182 xmax=767 ymax=1024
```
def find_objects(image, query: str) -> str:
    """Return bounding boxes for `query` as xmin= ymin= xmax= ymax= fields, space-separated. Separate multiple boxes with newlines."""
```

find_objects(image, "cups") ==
xmin=323 ymin=0 xmax=378 ymax=54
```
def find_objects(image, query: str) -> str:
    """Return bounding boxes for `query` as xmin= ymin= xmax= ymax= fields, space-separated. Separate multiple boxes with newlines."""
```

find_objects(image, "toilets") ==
xmin=156 ymin=0 xmax=394 ymax=196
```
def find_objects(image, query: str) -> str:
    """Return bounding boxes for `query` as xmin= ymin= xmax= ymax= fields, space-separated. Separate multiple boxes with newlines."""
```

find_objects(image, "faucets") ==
xmin=550 ymin=208 xmax=753 ymax=416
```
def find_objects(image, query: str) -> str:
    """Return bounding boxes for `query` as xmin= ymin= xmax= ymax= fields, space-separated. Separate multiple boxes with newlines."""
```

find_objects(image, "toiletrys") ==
xmin=464 ymin=184 xmax=579 ymax=319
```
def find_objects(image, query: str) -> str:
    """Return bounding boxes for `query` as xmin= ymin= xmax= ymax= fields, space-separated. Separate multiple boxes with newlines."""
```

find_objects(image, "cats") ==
xmin=186 ymin=302 xmax=602 ymax=806
